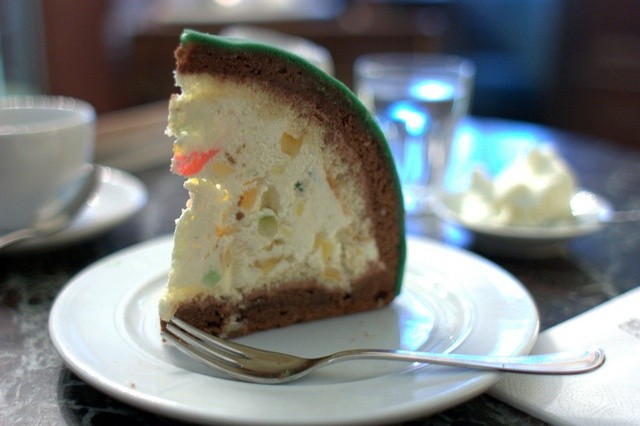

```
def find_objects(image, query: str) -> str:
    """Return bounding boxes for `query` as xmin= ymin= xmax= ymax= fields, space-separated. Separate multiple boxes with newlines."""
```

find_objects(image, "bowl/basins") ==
xmin=0 ymin=94 xmax=97 ymax=233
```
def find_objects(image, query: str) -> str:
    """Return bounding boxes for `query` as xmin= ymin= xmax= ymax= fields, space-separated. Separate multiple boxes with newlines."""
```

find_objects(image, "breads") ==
xmin=157 ymin=27 xmax=407 ymax=339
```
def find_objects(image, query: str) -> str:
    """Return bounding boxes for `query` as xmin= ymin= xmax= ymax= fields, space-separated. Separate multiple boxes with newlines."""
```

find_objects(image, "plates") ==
xmin=0 ymin=164 xmax=148 ymax=252
xmin=429 ymin=189 xmax=612 ymax=253
xmin=46 ymin=232 xmax=541 ymax=426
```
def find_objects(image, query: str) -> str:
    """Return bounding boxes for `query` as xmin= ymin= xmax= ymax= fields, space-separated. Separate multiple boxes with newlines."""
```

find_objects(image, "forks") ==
xmin=160 ymin=316 xmax=606 ymax=384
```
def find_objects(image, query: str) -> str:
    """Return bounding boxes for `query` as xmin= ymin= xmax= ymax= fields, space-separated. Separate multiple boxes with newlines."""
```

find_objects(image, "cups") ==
xmin=352 ymin=50 xmax=476 ymax=237
